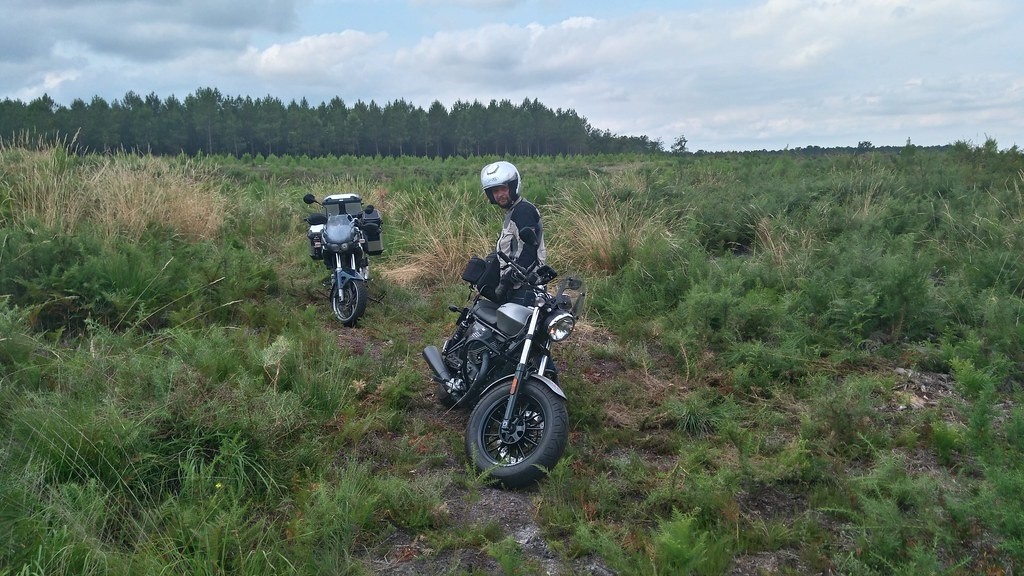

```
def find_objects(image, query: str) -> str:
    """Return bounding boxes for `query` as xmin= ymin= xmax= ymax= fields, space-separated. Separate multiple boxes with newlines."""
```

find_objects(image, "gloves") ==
xmin=495 ymin=277 xmax=514 ymax=307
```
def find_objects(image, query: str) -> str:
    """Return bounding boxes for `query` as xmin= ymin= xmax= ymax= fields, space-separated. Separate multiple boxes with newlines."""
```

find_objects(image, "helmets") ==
xmin=481 ymin=161 xmax=522 ymax=204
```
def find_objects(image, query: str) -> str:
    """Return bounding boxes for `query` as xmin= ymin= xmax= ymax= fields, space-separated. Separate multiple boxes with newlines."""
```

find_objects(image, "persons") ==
xmin=481 ymin=161 xmax=559 ymax=422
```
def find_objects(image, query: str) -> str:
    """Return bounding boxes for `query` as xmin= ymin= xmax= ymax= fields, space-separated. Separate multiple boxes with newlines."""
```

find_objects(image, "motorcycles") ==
xmin=303 ymin=194 xmax=384 ymax=326
xmin=422 ymin=251 xmax=585 ymax=490
